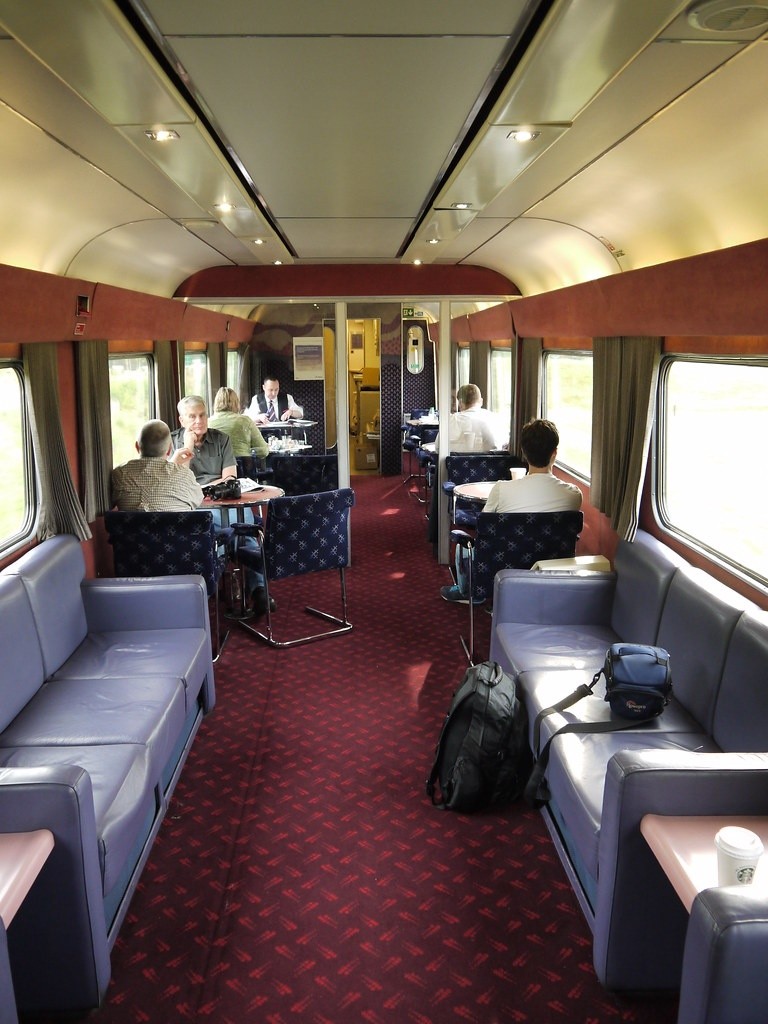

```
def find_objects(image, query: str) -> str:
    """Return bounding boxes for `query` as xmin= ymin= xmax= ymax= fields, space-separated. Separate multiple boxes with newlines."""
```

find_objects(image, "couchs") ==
xmin=489 ymin=528 xmax=768 ymax=991
xmin=0 ymin=533 xmax=216 ymax=1008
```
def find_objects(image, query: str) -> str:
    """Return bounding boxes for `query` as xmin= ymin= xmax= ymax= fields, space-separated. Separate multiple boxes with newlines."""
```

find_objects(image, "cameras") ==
xmin=201 ymin=479 xmax=242 ymax=501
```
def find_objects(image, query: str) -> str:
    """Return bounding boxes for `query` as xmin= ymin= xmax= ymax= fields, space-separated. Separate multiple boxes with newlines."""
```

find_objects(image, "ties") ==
xmin=269 ymin=400 xmax=277 ymax=422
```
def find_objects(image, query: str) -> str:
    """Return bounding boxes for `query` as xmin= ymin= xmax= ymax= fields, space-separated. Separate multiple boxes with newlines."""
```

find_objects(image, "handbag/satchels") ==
xmin=605 ymin=643 xmax=674 ymax=721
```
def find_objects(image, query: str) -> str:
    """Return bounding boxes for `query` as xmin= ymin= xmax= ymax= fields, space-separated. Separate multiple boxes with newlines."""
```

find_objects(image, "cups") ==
xmin=464 ymin=433 xmax=474 ymax=448
xmin=510 ymin=468 xmax=526 ymax=480
xmin=713 ymin=826 xmax=764 ymax=887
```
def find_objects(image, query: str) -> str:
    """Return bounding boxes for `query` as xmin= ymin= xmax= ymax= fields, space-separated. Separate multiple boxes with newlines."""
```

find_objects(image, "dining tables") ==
xmin=196 ymin=485 xmax=285 ymax=620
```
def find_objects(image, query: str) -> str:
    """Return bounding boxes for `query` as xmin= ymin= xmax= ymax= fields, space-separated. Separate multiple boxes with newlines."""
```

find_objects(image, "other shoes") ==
xmin=249 ymin=590 xmax=278 ymax=612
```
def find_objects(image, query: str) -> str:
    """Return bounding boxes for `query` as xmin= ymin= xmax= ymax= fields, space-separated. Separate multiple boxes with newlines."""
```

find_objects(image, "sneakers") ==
xmin=440 ymin=584 xmax=487 ymax=605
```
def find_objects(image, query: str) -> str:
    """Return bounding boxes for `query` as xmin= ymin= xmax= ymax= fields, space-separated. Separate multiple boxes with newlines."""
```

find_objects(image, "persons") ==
xmin=440 ymin=419 xmax=583 ymax=616
xmin=110 ymin=418 xmax=204 ymax=512
xmin=245 ymin=377 xmax=304 ymax=439
xmin=434 ymin=384 xmax=503 ymax=456
xmin=207 ymin=387 xmax=270 ymax=459
xmin=168 ymin=395 xmax=278 ymax=612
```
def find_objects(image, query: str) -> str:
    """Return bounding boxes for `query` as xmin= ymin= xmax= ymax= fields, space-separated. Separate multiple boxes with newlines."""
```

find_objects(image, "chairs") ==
xmin=233 ymin=409 xmax=439 ymax=523
xmin=443 ymin=455 xmax=522 ymax=585
xmin=103 ymin=510 xmax=235 ymax=663
xmin=230 ymin=487 xmax=356 ymax=650
xmin=450 ymin=510 xmax=583 ymax=667
xmin=272 ymin=455 xmax=339 ymax=497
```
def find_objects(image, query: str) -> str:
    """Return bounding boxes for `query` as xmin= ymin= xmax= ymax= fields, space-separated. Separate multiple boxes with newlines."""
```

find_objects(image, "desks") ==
xmin=255 ymin=420 xmax=318 ymax=444
xmin=452 ymin=481 xmax=498 ymax=504
xmin=422 ymin=442 xmax=436 ymax=454
xmin=266 ymin=444 xmax=312 ymax=456
xmin=406 ymin=419 xmax=439 ymax=504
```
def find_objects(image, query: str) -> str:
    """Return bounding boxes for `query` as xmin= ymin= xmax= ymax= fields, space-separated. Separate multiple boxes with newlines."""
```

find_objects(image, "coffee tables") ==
xmin=639 ymin=814 xmax=768 ymax=917
xmin=0 ymin=830 xmax=54 ymax=929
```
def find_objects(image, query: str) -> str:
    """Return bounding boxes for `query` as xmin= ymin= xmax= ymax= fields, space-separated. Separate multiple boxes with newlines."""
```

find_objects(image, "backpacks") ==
xmin=427 ymin=661 xmax=526 ymax=816
